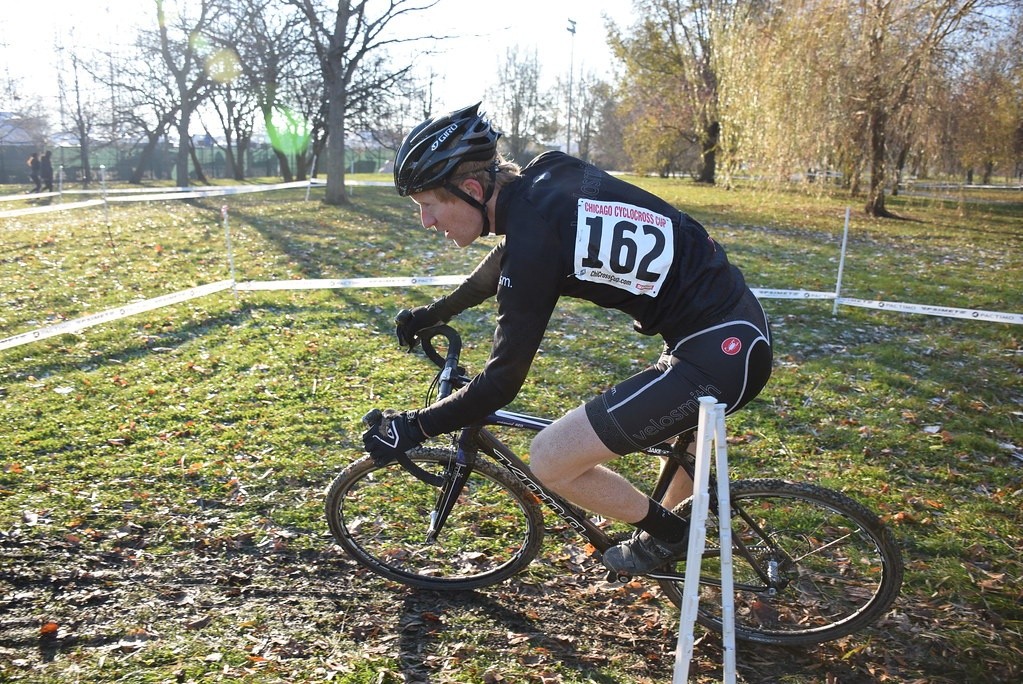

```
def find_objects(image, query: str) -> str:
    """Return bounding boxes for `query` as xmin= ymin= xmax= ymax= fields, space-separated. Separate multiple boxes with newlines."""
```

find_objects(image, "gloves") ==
xmin=395 ymin=301 xmax=455 ymax=346
xmin=363 ymin=409 xmax=427 ymax=466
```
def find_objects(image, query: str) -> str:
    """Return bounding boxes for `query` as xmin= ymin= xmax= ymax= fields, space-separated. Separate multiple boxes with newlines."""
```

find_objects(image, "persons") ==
xmin=39 ymin=151 xmax=53 ymax=201
xmin=28 ymin=152 xmax=41 ymax=198
xmin=359 ymin=102 xmax=772 ymax=574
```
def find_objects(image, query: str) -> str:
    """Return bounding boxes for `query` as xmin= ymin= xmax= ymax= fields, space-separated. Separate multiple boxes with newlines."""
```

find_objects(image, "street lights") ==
xmin=566 ymin=18 xmax=576 ymax=155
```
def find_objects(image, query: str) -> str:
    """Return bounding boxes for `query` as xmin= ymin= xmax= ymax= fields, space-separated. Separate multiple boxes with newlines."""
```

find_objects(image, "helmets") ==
xmin=394 ymin=101 xmax=502 ymax=197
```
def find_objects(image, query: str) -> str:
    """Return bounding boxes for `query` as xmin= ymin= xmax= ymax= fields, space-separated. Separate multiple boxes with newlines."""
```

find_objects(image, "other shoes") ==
xmin=602 ymin=525 xmax=689 ymax=576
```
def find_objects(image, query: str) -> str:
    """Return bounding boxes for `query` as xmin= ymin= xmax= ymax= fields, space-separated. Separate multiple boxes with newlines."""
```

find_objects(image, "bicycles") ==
xmin=322 ymin=324 xmax=905 ymax=649
